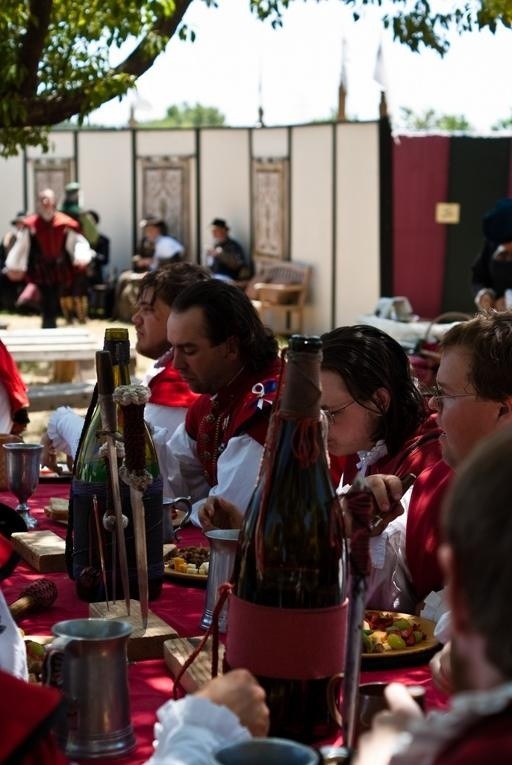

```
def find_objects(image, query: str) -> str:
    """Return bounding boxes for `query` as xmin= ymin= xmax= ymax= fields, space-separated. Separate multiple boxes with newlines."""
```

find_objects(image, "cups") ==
xmin=162 ymin=497 xmax=193 ymax=541
xmin=212 ymin=735 xmax=320 ymax=765
xmin=46 ymin=620 xmax=140 ymax=763
xmin=202 ymin=529 xmax=248 ymax=634
xmin=68 ymin=327 xmax=163 ymax=601
xmin=355 ymin=681 xmax=427 ymax=746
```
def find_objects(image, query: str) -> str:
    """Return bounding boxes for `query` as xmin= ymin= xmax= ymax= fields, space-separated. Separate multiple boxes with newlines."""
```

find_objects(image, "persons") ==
xmin=2 ymin=343 xmax=269 ymax=764
xmin=471 ymin=194 xmax=509 ymax=318
xmin=37 ymin=260 xmax=510 ymax=763
xmin=2 ymin=183 xmax=253 ymax=329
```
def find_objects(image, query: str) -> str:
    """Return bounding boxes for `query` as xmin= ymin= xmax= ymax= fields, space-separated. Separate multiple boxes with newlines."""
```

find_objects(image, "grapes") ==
xmin=387 ymin=634 xmax=406 ymax=649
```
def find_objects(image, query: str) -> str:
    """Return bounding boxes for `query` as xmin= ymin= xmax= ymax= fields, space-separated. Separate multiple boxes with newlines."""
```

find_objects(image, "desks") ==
xmin=0 ymin=326 xmax=136 ymax=382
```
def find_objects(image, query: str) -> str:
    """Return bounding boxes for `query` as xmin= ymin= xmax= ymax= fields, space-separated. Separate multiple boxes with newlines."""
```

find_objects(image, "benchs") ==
xmin=25 ymin=383 xmax=95 ymax=413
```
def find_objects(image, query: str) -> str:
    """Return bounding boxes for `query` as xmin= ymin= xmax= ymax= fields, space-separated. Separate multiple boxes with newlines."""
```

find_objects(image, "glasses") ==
xmin=431 ymin=385 xmax=488 ymax=404
xmin=321 ymin=400 xmax=367 ymax=426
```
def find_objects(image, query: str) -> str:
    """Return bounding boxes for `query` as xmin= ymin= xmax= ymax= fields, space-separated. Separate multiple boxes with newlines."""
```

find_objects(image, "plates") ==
xmin=162 ymin=563 xmax=211 ymax=587
xmin=41 ymin=462 xmax=72 ymax=483
xmin=357 ymin=607 xmax=438 ymax=659
xmin=58 ymin=518 xmax=70 ymax=525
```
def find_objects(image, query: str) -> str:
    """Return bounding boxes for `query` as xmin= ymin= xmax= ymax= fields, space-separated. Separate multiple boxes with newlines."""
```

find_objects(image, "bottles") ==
xmin=226 ymin=335 xmax=346 ymax=749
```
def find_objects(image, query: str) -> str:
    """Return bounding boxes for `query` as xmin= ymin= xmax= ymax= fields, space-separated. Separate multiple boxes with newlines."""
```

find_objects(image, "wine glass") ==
xmin=3 ymin=441 xmax=45 ymax=529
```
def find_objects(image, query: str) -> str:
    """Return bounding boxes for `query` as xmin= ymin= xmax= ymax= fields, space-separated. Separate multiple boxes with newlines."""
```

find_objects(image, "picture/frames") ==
xmin=32 ymin=156 xmax=73 ymax=214
xmin=250 ymin=158 xmax=292 ymax=262
xmin=141 ymin=155 xmax=192 ymax=262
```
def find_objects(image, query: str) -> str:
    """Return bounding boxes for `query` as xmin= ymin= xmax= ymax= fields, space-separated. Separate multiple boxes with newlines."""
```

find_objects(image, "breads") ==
xmin=44 ymin=496 xmax=70 ymax=521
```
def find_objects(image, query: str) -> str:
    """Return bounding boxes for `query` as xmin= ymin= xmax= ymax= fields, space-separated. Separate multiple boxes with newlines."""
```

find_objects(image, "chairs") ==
xmin=232 ymin=261 xmax=312 ymax=337
